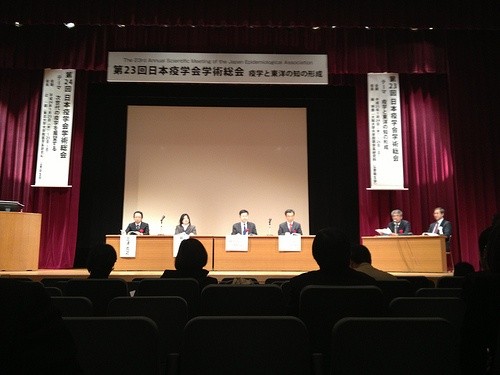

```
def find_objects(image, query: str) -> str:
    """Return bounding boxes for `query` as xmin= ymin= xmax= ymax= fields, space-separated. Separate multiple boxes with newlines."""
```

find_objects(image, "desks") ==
xmin=105 ymin=234 xmax=448 ymax=273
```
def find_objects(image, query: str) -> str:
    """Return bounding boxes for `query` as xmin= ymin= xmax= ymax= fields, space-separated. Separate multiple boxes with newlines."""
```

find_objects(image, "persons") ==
xmin=125 ymin=211 xmax=149 ymax=235
xmin=428 ymin=208 xmax=452 ymax=252
xmin=278 ymin=209 xmax=302 ymax=235
xmin=282 ymin=228 xmax=399 ymax=318
xmin=454 ymin=232 xmax=500 ymax=280
xmin=231 ymin=210 xmax=258 ymax=236
xmin=87 ymin=244 xmax=117 ymax=279
xmin=381 ymin=210 xmax=411 ymax=235
xmin=161 ymin=237 xmax=218 ymax=285
xmin=175 ymin=214 xmax=197 ymax=236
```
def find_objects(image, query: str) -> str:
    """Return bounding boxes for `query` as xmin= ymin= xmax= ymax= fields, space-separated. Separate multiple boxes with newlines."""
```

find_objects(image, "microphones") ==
xmin=161 ymin=215 xmax=165 ymax=221
xmin=268 ymin=218 xmax=272 ymax=225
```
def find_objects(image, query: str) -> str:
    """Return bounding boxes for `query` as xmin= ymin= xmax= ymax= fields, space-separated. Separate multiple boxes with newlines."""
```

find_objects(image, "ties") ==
xmin=289 ymin=224 xmax=292 ymax=231
xmin=243 ymin=224 xmax=246 ymax=235
xmin=137 ymin=225 xmax=139 ymax=229
xmin=434 ymin=222 xmax=438 ymax=233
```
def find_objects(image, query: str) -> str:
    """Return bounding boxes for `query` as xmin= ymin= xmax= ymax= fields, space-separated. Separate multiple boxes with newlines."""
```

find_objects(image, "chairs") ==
xmin=1 ymin=277 xmax=466 ymax=375
